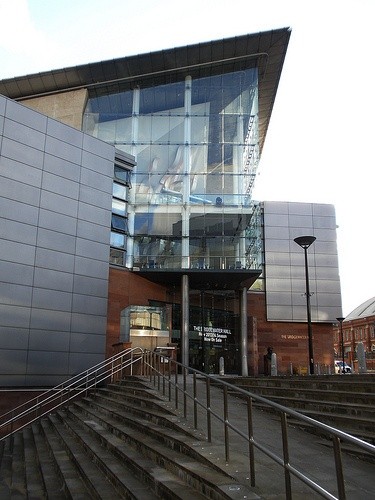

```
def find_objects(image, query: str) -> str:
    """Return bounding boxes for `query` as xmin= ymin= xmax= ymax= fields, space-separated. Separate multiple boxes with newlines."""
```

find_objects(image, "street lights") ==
xmin=335 ymin=317 xmax=346 ymax=373
xmin=293 ymin=234 xmax=317 ymax=375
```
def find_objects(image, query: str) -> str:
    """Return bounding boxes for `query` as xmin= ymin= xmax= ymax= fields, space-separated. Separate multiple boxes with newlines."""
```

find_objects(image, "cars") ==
xmin=335 ymin=361 xmax=351 ymax=373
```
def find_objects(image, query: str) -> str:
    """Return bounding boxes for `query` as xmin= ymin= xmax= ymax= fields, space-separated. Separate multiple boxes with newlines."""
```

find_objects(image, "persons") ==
xmin=264 ymin=347 xmax=273 ymax=360
